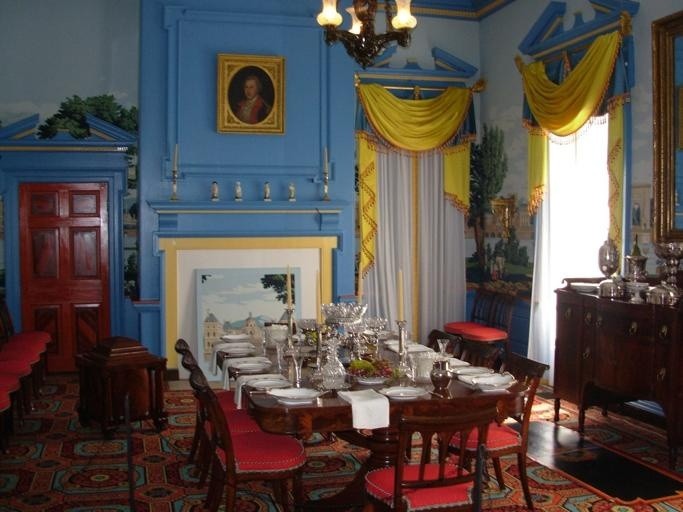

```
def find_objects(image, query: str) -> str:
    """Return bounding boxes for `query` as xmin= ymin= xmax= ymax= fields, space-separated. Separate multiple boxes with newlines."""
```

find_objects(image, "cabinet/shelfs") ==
xmin=553 ymin=270 xmax=683 ymax=471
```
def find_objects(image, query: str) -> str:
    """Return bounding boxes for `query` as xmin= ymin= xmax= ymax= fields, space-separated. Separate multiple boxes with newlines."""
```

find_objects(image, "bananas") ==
xmin=350 ymin=360 xmax=374 ymax=371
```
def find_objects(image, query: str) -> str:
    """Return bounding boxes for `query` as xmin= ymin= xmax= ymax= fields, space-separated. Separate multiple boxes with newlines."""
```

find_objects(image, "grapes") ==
xmin=349 ymin=368 xmax=369 ymax=378
xmin=373 ymin=360 xmax=400 ymax=385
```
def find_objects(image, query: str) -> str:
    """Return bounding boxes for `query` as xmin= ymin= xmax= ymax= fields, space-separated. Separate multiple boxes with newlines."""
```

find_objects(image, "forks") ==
xmin=219 ymin=333 xmax=252 ymax=341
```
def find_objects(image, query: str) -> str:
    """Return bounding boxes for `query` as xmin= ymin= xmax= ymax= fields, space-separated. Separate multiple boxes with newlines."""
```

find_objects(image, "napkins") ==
xmin=338 ymin=388 xmax=391 ymax=429
xmin=210 ymin=340 xmax=255 ymax=377
xmin=234 ymin=371 xmax=287 ymax=410
xmin=222 ymin=355 xmax=272 ymax=394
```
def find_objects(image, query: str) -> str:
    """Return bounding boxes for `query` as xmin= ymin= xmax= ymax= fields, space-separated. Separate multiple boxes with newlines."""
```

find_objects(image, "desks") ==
xmin=210 ymin=327 xmax=512 ymax=512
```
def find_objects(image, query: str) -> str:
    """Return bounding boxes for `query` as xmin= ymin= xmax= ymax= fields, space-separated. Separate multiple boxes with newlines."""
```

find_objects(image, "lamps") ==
xmin=314 ymin=0 xmax=419 ymax=70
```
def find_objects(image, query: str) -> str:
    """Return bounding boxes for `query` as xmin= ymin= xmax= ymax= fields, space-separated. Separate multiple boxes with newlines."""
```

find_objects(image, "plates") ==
xmin=228 ymin=357 xmax=272 ymax=373
xmin=216 ymin=343 xmax=257 ymax=357
xmin=354 ymin=377 xmax=382 ymax=385
xmin=376 ymin=384 xmax=430 ymax=402
xmin=383 ymin=336 xmax=518 ymax=389
xmin=570 ymin=281 xmax=601 ymax=295
xmin=243 ymin=376 xmax=293 ymax=391
xmin=268 ymin=387 xmax=322 ymax=407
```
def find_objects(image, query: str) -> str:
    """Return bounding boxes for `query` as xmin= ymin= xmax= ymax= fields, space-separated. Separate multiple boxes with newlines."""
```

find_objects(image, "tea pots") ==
xmin=648 ymin=284 xmax=671 ymax=306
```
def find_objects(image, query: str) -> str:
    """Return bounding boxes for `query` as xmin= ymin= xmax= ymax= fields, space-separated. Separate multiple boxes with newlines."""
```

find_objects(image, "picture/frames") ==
xmin=218 ymin=53 xmax=285 ymax=135
xmin=649 ymin=10 xmax=683 ymax=249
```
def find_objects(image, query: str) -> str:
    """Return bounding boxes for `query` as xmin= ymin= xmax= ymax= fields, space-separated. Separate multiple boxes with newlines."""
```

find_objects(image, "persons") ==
xmin=233 ymin=75 xmax=273 ymax=126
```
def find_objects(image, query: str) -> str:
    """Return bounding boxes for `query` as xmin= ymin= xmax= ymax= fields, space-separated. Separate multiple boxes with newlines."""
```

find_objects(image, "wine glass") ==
xmin=625 ymin=280 xmax=649 ymax=306
xmin=260 ymin=327 xmax=305 ymax=380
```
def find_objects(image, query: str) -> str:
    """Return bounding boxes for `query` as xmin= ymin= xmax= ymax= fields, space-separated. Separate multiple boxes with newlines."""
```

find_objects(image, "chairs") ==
xmin=363 ymin=405 xmax=503 ymax=512
xmin=174 ymin=338 xmax=305 ymax=511
xmin=443 ymin=285 xmax=495 ymax=362
xmin=0 ymin=297 xmax=53 ymax=445
xmin=426 ymin=328 xmax=551 ymax=512
xmin=463 ymin=290 xmax=519 ymax=373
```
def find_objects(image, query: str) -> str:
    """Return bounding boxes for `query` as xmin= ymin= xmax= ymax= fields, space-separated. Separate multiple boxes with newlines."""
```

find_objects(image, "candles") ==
xmin=324 ymin=145 xmax=328 ymax=171
xmin=314 ymin=270 xmax=325 ymax=327
xmin=287 ymin=262 xmax=292 ymax=307
xmin=398 ymin=267 xmax=403 ymax=321
xmin=173 ymin=143 xmax=179 ymax=169
xmin=356 ymin=259 xmax=363 ymax=306
